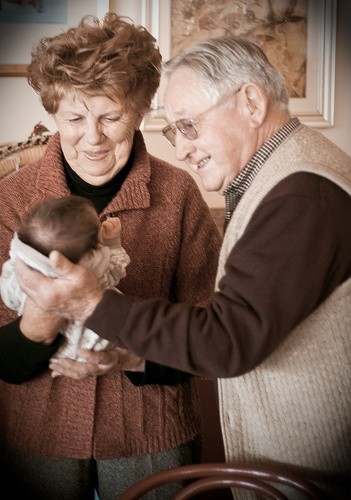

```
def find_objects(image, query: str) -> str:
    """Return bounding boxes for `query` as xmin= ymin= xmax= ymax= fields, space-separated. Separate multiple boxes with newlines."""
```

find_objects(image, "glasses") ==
xmin=161 ymin=86 xmax=242 ymax=147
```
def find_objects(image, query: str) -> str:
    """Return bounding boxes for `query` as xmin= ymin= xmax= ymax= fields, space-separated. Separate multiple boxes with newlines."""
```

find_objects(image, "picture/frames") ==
xmin=141 ymin=0 xmax=335 ymax=128
xmin=0 ymin=0 xmax=116 ymax=77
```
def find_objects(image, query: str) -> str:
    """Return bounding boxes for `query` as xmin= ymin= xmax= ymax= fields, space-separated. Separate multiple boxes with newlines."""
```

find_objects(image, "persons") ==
xmin=159 ymin=34 xmax=351 ymax=500
xmin=1 ymin=15 xmax=224 ymax=500
xmin=0 ymin=196 xmax=132 ymax=362
